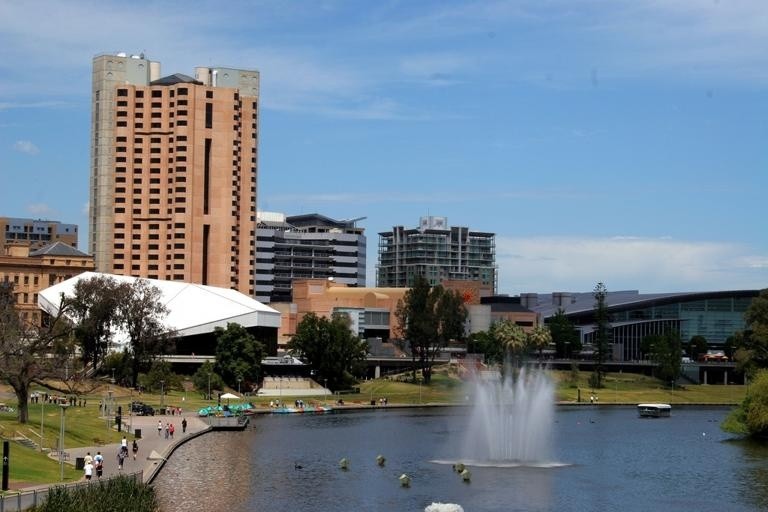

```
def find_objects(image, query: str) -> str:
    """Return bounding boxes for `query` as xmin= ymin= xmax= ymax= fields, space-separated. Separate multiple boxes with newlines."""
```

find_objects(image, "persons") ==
xmin=120 ymin=435 xmax=129 ymax=455
xmin=94 ymin=458 xmax=105 ymax=479
xmin=84 ymin=451 xmax=94 ymax=465
xmin=83 ymin=461 xmax=94 ymax=480
xmin=379 ymin=395 xmax=388 ymax=406
xmin=131 ymin=440 xmax=139 ymax=461
xmin=247 ymin=399 xmax=304 ymax=409
xmin=98 ymin=400 xmax=103 ymax=410
xmin=115 ymin=446 xmax=126 ymax=470
xmin=335 ymin=398 xmax=343 ymax=406
xmin=30 ymin=392 xmax=88 ymax=408
xmin=93 ymin=451 xmax=104 ymax=460
xmin=139 ymin=401 xmax=190 ymax=441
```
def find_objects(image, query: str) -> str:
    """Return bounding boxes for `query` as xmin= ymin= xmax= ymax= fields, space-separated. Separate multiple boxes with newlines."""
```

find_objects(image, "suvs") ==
xmin=128 ymin=401 xmax=154 ymax=416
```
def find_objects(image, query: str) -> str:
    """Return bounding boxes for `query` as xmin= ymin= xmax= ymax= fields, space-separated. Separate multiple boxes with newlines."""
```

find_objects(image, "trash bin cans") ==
xmin=160 ymin=409 xmax=165 ymax=415
xmin=371 ymin=400 xmax=375 ymax=405
xmin=116 ymin=417 xmax=119 ymax=423
xmin=135 ymin=429 xmax=141 ymax=438
xmin=76 ymin=458 xmax=84 ymax=470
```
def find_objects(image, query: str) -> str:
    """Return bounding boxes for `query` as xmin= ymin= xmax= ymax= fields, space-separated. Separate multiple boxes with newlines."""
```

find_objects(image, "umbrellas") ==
xmin=220 ymin=391 xmax=241 ymax=406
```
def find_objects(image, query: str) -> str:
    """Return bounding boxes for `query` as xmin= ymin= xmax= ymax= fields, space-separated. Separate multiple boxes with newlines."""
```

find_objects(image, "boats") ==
xmin=637 ymin=404 xmax=672 ymax=418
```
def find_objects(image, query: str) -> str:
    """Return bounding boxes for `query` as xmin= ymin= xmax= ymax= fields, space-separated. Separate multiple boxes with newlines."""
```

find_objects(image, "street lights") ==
xmin=418 ymin=378 xmax=424 ymax=402
xmin=671 ymin=380 xmax=674 ymax=403
xmin=322 ymin=378 xmax=330 ymax=403
xmin=108 ymin=390 xmax=114 ymax=431
xmin=129 ymin=387 xmax=135 ymax=413
xmin=370 ymin=378 xmax=374 ymax=400
xmin=59 ymin=403 xmax=70 ymax=481
xmin=206 ymin=372 xmax=213 ymax=406
xmin=236 ymin=379 xmax=244 ymax=414
xmin=160 ymin=380 xmax=165 ymax=405
xmin=39 ymin=392 xmax=47 ymax=451
